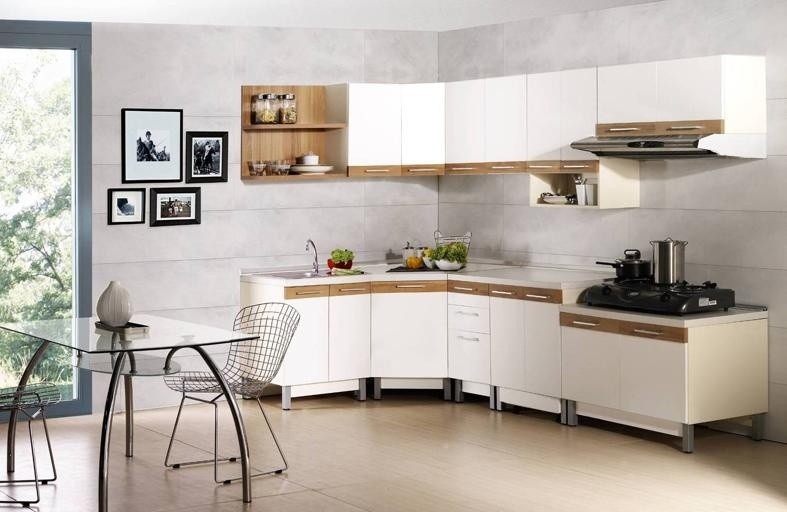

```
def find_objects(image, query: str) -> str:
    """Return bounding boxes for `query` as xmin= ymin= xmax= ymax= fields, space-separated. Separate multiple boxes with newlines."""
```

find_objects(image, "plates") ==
xmin=289 ymin=165 xmax=334 ymax=176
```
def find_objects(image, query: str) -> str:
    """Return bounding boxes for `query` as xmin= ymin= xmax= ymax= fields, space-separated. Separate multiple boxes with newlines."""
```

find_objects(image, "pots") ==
xmin=648 ymin=237 xmax=689 ymax=284
xmin=594 ymin=248 xmax=651 ymax=280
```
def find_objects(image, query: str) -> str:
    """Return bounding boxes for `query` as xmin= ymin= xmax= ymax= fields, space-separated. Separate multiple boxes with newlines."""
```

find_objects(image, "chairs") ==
xmin=164 ymin=301 xmax=303 ymax=488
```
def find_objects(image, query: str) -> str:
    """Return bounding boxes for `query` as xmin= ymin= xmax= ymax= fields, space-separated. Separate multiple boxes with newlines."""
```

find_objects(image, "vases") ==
xmin=95 ymin=277 xmax=135 ymax=327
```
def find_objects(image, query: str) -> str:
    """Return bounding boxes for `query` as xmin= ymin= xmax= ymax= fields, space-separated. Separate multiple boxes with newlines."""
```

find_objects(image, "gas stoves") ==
xmin=582 ymin=282 xmax=736 ymax=317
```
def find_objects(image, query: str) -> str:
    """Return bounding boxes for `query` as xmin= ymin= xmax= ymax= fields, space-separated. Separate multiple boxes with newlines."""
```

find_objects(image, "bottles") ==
xmin=401 ymin=247 xmax=414 ymax=263
xmin=255 ymin=93 xmax=296 ymax=125
xmin=417 ymin=247 xmax=429 ymax=257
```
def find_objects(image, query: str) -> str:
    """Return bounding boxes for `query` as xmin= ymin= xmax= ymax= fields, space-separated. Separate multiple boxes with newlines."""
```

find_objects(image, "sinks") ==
xmin=268 ymin=272 xmax=322 ymax=280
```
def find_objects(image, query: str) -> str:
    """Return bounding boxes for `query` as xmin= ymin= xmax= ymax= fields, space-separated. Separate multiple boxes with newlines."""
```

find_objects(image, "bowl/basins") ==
xmin=540 ymin=196 xmax=567 ymax=205
xmin=421 ymin=257 xmax=461 ymax=270
xmin=327 ymin=258 xmax=353 ymax=270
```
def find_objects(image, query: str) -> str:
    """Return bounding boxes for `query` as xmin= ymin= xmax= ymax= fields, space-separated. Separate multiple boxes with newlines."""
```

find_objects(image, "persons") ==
xmin=166 ymin=197 xmax=183 ymax=216
xmin=143 ymin=131 xmax=159 ymax=161
xmin=194 ymin=141 xmax=216 ymax=174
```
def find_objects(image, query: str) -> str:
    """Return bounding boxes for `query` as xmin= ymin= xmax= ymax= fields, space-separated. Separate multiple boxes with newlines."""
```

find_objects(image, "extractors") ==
xmin=571 ymin=133 xmax=716 ymax=158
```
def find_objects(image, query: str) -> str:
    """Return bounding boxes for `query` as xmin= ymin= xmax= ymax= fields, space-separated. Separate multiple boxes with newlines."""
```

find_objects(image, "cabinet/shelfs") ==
xmin=488 ymin=280 xmax=569 ymax=426
xmin=527 ymin=66 xmax=643 ymax=209
xmin=239 ymin=81 xmax=345 ymax=184
xmin=326 ymin=80 xmax=444 ymax=179
xmin=448 ymin=274 xmax=497 ymax=412
xmin=370 ymin=272 xmax=454 ymax=401
xmin=445 ymin=73 xmax=527 ymax=174
xmin=596 ymin=52 xmax=767 ymax=159
xmin=240 ymin=276 xmax=372 ymax=412
xmin=558 ymin=302 xmax=772 ymax=454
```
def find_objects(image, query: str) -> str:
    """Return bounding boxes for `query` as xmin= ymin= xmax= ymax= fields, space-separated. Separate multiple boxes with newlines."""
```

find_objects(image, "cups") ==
xmin=250 ymin=160 xmax=291 ymax=176
xmin=303 ymin=151 xmax=319 ymax=166
xmin=575 ymin=184 xmax=597 ymax=206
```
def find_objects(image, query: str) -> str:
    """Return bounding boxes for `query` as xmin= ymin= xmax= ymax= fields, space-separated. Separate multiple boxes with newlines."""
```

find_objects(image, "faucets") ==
xmin=305 ymin=239 xmax=318 ymax=273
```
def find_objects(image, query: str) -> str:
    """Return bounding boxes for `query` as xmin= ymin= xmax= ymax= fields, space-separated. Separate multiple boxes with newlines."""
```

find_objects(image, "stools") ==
xmin=0 ymin=380 xmax=62 ymax=507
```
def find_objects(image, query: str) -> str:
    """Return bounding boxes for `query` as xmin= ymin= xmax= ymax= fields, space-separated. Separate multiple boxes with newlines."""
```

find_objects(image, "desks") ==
xmin=0 ymin=310 xmax=262 ymax=512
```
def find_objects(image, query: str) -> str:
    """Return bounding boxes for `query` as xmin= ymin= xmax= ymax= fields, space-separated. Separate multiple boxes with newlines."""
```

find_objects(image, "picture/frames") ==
xmin=118 ymin=105 xmax=183 ymax=187
xmin=107 ymin=186 xmax=144 ymax=227
xmin=148 ymin=186 xmax=203 ymax=228
xmin=185 ymin=130 xmax=228 ymax=183
